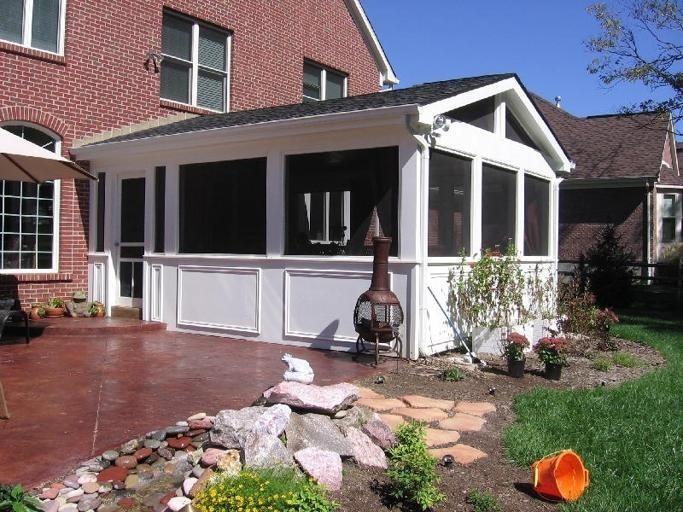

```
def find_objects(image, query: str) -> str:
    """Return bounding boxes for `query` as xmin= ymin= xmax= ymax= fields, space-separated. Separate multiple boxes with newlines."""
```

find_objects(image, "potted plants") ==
xmin=28 ymin=297 xmax=65 ymax=320
xmin=91 ymin=301 xmax=105 ymax=317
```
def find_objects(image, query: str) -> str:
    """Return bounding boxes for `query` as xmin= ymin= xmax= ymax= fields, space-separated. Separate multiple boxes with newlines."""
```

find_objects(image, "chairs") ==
xmin=0 ymin=275 xmax=29 ymax=345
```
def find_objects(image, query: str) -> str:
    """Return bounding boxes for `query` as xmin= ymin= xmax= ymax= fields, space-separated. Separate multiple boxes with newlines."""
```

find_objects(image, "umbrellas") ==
xmin=0 ymin=125 xmax=100 ymax=186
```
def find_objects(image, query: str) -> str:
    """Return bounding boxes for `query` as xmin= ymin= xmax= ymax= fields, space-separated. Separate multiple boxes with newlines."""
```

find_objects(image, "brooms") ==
xmin=427 ymin=285 xmax=482 ymax=364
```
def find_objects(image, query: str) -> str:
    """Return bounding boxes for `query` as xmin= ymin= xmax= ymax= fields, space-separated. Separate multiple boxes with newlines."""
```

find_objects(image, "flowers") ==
xmin=500 ymin=331 xmax=571 ymax=367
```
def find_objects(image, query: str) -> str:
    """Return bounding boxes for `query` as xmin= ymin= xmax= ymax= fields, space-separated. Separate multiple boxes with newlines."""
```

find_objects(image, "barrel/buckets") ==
xmin=531 ymin=449 xmax=591 ymax=505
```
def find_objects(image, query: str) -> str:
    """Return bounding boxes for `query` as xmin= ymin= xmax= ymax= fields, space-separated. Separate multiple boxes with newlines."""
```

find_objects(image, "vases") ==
xmin=506 ymin=359 xmax=561 ymax=381
